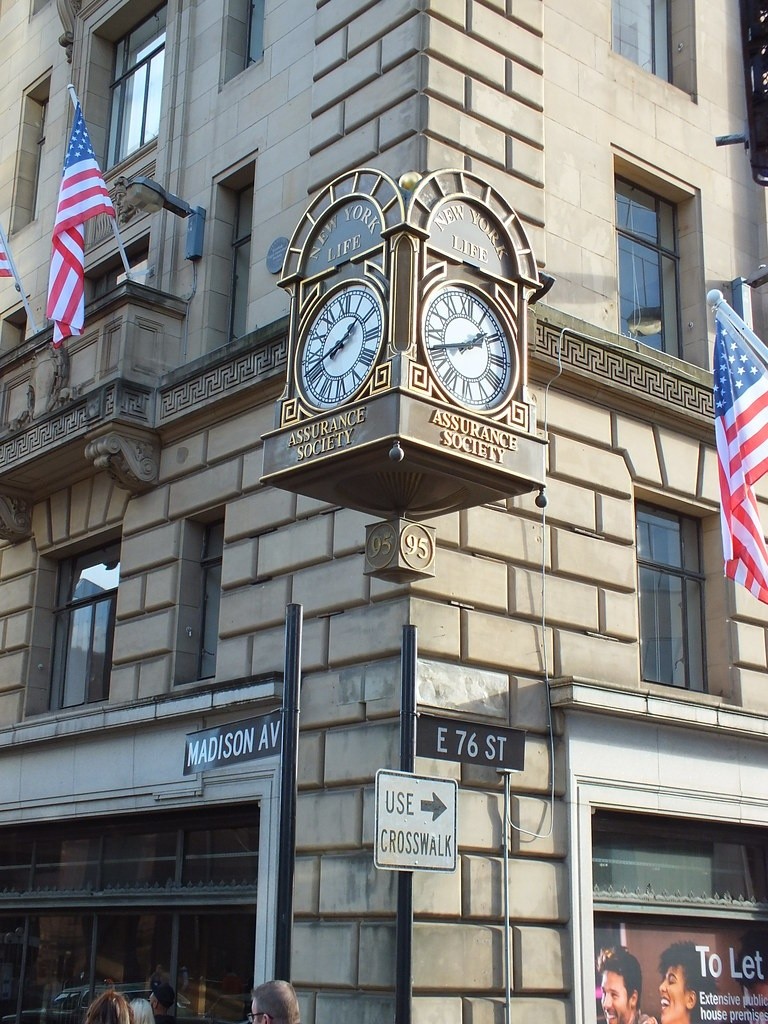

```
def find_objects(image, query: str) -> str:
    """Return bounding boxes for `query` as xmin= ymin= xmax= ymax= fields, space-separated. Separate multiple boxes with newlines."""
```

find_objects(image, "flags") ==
xmin=47 ymin=101 xmax=116 ymax=349
xmin=713 ymin=310 xmax=768 ymax=605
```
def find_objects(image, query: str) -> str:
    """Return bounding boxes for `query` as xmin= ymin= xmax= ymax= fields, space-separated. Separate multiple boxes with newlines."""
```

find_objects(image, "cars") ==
xmin=2 ymin=983 xmax=253 ymax=1024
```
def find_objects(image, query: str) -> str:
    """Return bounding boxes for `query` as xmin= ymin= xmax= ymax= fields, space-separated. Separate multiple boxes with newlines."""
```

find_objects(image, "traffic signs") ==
xmin=373 ymin=765 xmax=459 ymax=873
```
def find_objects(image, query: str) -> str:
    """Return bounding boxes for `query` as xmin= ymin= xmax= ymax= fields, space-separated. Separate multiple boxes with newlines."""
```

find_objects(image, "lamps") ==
xmin=627 ymin=305 xmax=662 ymax=337
xmin=124 ymin=177 xmax=205 ymax=262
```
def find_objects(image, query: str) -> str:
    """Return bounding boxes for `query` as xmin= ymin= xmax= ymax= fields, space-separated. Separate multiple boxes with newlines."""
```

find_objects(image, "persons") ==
xmin=150 ymin=963 xmax=162 ymax=989
xmin=247 ymin=980 xmax=301 ymax=1024
xmin=734 ymin=927 xmax=768 ymax=1024
xmin=114 ymin=175 xmax=136 ymax=223
xmin=659 ymin=940 xmax=719 ymax=1024
xmin=222 ymin=964 xmax=242 ymax=994
xmin=596 ymin=945 xmax=658 ymax=1024
xmin=82 ymin=981 xmax=178 ymax=1024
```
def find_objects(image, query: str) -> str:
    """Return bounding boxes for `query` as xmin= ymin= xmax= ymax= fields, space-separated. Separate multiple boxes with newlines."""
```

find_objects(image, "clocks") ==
xmin=299 ymin=283 xmax=385 ymax=411
xmin=422 ymin=283 xmax=513 ymax=413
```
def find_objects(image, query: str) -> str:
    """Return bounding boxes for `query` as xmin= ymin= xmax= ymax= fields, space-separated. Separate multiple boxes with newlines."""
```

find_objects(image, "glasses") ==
xmin=247 ymin=1012 xmax=273 ymax=1022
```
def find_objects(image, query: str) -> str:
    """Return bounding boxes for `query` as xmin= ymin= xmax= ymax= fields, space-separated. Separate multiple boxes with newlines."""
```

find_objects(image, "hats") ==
xmin=151 ymin=981 xmax=175 ymax=1010
